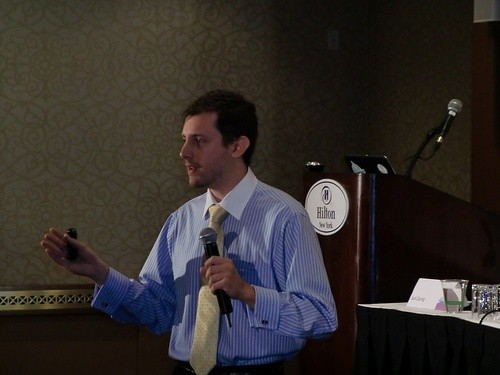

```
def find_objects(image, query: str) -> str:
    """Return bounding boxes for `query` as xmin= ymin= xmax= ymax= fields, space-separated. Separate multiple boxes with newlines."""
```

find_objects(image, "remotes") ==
xmin=67 ymin=228 xmax=79 ymax=261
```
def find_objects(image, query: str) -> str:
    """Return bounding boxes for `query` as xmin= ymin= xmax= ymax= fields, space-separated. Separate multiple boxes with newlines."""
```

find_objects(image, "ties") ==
xmin=189 ymin=205 xmax=229 ymax=375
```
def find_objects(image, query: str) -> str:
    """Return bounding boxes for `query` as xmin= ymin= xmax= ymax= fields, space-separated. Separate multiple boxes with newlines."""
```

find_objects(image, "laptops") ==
xmin=344 ymin=156 xmax=396 ymax=175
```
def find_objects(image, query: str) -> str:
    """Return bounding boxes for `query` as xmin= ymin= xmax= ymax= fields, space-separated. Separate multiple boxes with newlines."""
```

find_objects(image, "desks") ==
xmin=354 ymin=301 xmax=500 ymax=375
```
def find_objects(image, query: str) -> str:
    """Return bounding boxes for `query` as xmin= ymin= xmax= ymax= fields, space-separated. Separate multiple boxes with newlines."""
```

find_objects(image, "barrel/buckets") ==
xmin=440 ymin=279 xmax=469 ymax=313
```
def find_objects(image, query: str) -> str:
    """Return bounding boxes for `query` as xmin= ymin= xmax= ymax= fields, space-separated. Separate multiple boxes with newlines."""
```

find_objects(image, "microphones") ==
xmin=200 ymin=227 xmax=233 ymax=329
xmin=434 ymin=99 xmax=463 ymax=151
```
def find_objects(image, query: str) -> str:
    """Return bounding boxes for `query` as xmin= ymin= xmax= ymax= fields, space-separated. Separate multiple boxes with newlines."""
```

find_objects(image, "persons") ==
xmin=41 ymin=91 xmax=338 ymax=375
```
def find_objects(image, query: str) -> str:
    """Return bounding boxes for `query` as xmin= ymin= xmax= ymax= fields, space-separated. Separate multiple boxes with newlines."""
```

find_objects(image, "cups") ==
xmin=471 ymin=283 xmax=500 ymax=322
